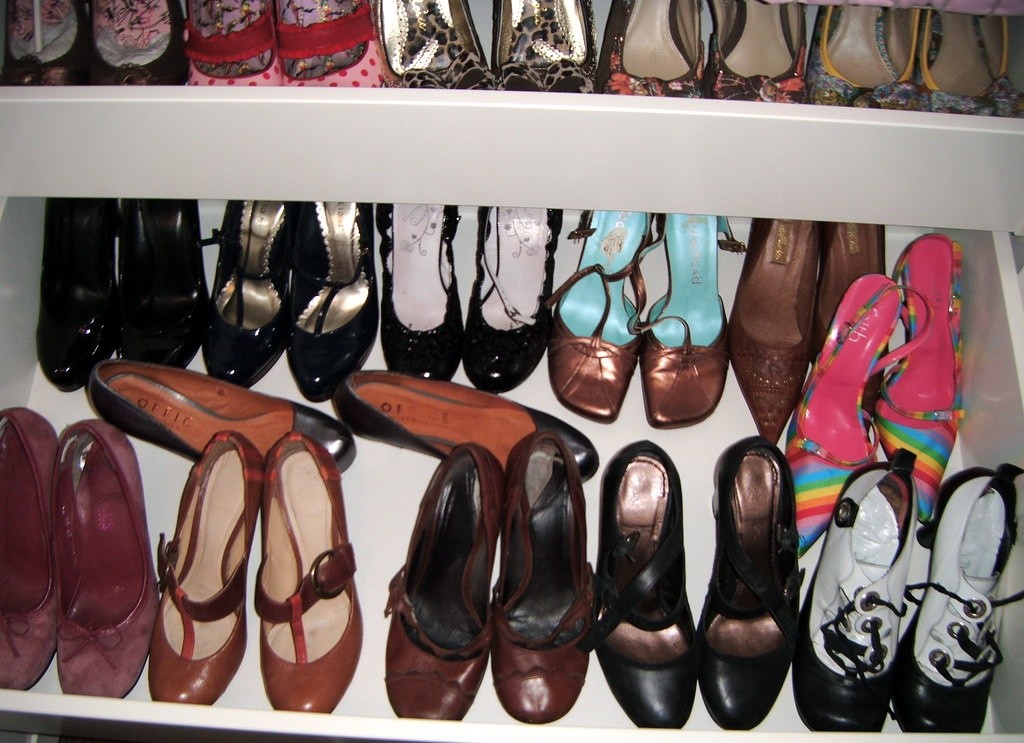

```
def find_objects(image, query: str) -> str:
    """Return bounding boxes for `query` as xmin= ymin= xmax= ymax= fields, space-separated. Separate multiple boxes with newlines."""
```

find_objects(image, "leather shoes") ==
xmin=51 ymin=418 xmax=160 ymax=698
xmin=792 ymin=461 xmax=908 ymax=732
xmin=696 ymin=436 xmax=807 ymax=730
xmin=34 ymin=197 xmax=889 ymax=446
xmin=384 ymin=441 xmax=506 ymax=721
xmin=254 ymin=430 xmax=364 ymax=713
xmin=332 ymin=370 xmax=599 ymax=485
xmin=88 ymin=359 xmax=357 ymax=477
xmin=149 ymin=429 xmax=265 ymax=705
xmin=575 ymin=441 xmax=695 ymax=729
xmin=890 ymin=462 xmax=1024 ymax=733
xmin=0 ymin=407 xmax=57 ymax=690
xmin=490 ymin=430 xmax=595 ymax=724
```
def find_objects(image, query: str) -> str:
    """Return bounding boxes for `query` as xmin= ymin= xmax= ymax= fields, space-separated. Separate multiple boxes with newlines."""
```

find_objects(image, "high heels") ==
xmin=872 ymin=233 xmax=965 ymax=527
xmin=785 ymin=272 xmax=933 ymax=560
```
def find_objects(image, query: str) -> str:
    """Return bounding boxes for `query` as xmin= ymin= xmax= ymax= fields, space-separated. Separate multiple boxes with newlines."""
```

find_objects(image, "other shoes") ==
xmin=0 ymin=0 xmax=1024 ymax=119
